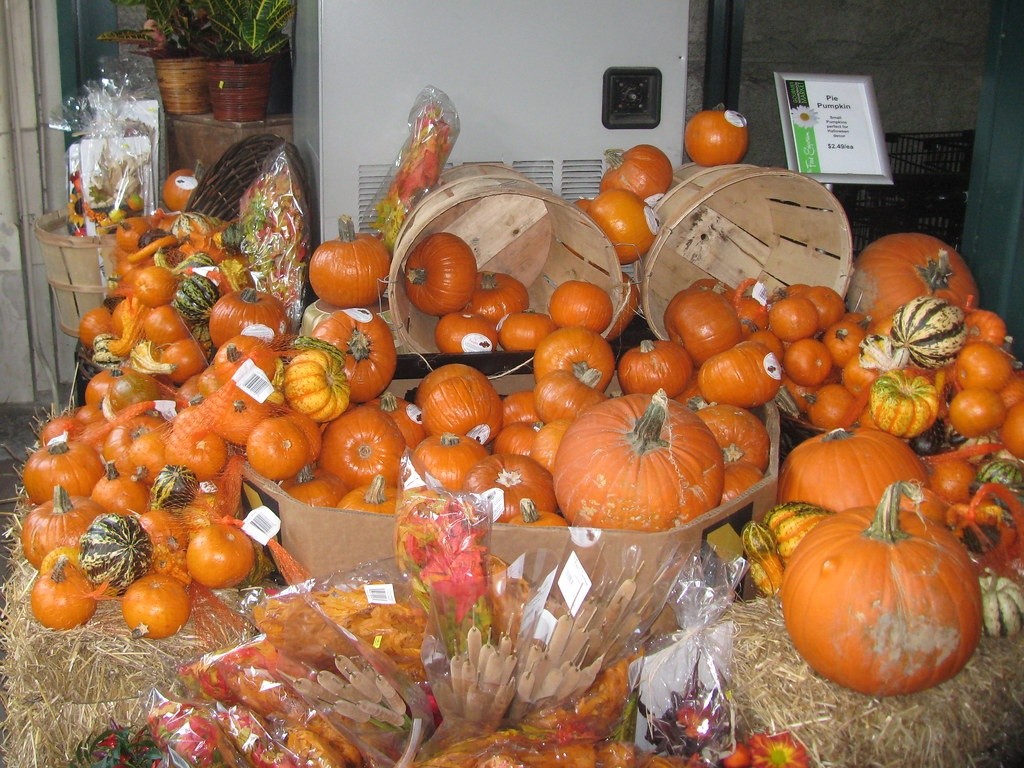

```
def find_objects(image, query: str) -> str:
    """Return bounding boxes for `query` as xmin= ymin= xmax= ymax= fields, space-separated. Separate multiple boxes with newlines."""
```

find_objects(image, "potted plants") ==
xmin=96 ymin=0 xmax=299 ymax=123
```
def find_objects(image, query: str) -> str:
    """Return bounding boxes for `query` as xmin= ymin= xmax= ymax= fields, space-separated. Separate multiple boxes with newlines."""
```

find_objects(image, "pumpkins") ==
xmin=22 ymin=144 xmax=1024 ymax=697
xmin=685 ymin=103 xmax=749 ymax=166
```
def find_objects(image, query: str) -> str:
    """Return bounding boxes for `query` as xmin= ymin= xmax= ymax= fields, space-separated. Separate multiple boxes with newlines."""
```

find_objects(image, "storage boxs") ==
xmin=834 ymin=129 xmax=974 ymax=257
